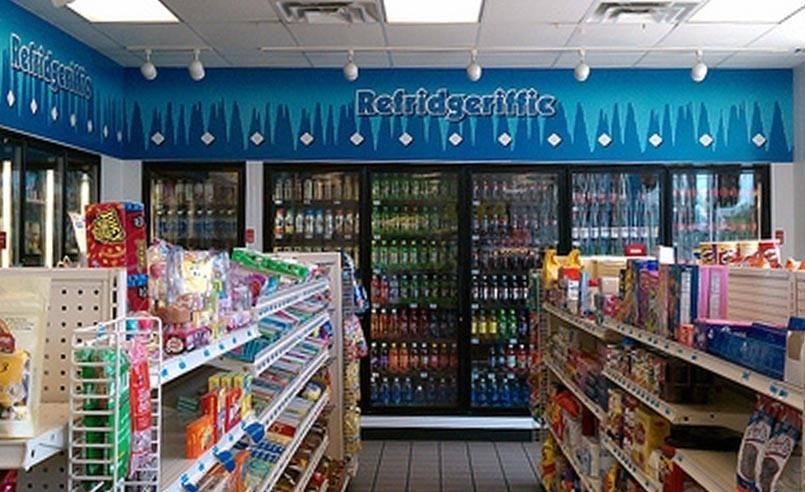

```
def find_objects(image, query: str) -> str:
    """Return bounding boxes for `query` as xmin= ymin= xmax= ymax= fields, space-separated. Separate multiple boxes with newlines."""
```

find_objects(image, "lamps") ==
xmin=573 ymin=49 xmax=590 ymax=81
xmin=341 ymin=49 xmax=360 ymax=82
xmin=465 ymin=50 xmax=482 ymax=82
xmin=141 ymin=44 xmax=206 ymax=82
xmin=690 ymin=50 xmax=709 ymax=82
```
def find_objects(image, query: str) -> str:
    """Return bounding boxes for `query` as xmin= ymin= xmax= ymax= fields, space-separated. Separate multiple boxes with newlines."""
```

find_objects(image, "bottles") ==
xmin=269 ymin=169 xmax=360 ymax=270
xmin=668 ymin=172 xmax=759 ymax=242
xmin=471 ymin=176 xmax=558 ymax=406
xmin=572 ymin=172 xmax=660 ymax=244
xmin=152 ymin=178 xmax=237 ymax=240
xmin=370 ymin=173 xmax=461 ymax=405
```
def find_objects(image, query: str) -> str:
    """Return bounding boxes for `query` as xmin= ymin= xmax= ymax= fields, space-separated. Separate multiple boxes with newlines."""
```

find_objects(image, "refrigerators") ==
xmin=140 ymin=162 xmax=243 ymax=252
xmin=0 ymin=136 xmax=101 ymax=269
xmin=663 ymin=163 xmax=773 ymax=264
xmin=469 ymin=165 xmax=567 ymax=415
xmin=567 ymin=164 xmax=666 ymax=255
xmin=364 ymin=164 xmax=469 ymax=415
xmin=265 ymin=163 xmax=366 ymax=413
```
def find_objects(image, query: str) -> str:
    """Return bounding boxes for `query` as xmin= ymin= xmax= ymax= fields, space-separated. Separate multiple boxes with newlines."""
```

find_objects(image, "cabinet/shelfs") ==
xmin=535 ymin=253 xmax=804 ymax=492
xmin=0 ymin=251 xmax=343 ymax=491
